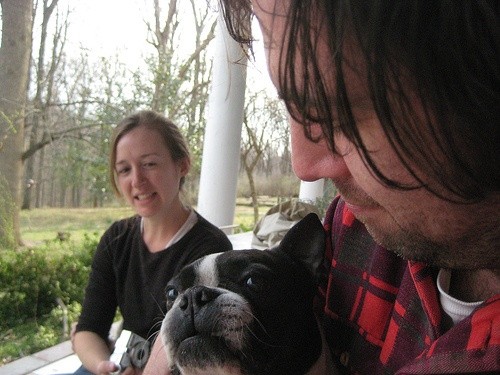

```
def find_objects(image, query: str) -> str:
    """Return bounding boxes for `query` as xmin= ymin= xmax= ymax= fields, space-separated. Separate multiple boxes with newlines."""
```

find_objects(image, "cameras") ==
xmin=108 ymin=329 xmax=150 ymax=375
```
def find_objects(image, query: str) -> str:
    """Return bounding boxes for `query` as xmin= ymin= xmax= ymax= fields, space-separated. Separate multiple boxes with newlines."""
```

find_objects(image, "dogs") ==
xmin=157 ymin=213 xmax=339 ymax=375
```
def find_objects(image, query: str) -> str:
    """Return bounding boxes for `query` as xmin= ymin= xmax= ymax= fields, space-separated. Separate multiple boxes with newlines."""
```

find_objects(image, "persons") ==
xmin=139 ymin=0 xmax=500 ymax=374
xmin=64 ymin=111 xmax=234 ymax=375
